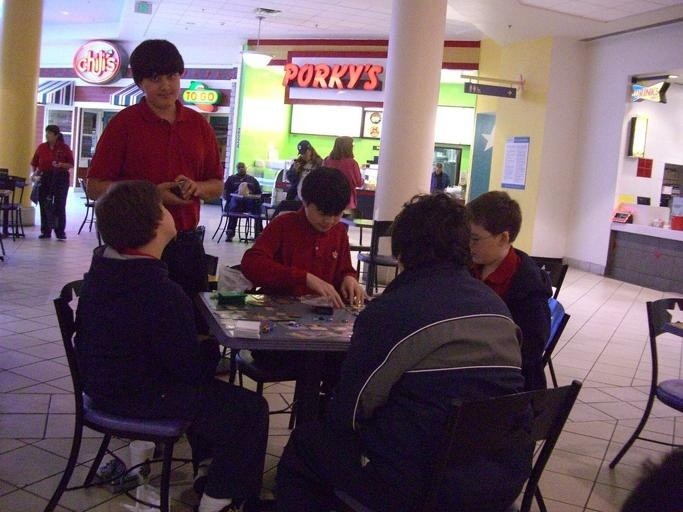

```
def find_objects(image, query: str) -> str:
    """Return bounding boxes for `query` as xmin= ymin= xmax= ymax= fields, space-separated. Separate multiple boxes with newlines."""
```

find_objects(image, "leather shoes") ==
xmin=225 ymin=236 xmax=232 ymax=242
xmin=39 ymin=233 xmax=51 ymax=238
xmin=57 ymin=234 xmax=66 ymax=239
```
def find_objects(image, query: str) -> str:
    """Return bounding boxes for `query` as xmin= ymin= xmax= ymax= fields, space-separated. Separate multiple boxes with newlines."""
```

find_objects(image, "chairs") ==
xmin=356 ymin=221 xmax=400 ymax=296
xmin=0 ymin=176 xmax=27 ymax=239
xmin=609 ymin=299 xmax=683 ymax=471
xmin=212 ymin=196 xmax=249 ymax=244
xmin=228 ymin=265 xmax=300 ymax=430
xmin=44 ymin=274 xmax=199 ymax=512
xmin=530 ymin=256 xmax=569 ymax=299
xmin=542 ymin=296 xmax=570 ymax=387
xmin=426 ymin=380 xmax=581 ymax=511
xmin=0 ymin=182 xmax=17 ymax=242
xmin=78 ymin=180 xmax=97 ymax=236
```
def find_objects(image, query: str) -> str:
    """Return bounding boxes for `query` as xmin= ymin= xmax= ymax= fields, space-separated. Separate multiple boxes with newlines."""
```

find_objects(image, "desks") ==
xmin=242 ymin=193 xmax=273 ymax=202
xmin=340 ymin=219 xmax=379 ymax=295
xmin=603 ymin=220 xmax=683 ymax=296
xmin=196 ymin=291 xmax=368 ymax=352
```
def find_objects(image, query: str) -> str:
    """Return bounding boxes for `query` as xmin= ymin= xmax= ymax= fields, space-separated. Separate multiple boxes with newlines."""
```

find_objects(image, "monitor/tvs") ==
xmin=630 ymin=115 xmax=649 ymax=159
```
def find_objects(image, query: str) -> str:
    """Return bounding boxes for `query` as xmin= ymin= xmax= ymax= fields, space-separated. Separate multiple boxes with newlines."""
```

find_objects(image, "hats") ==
xmin=297 ymin=140 xmax=310 ymax=155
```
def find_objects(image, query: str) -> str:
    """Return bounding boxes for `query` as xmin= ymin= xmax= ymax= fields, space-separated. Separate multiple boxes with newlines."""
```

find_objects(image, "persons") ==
xmin=240 ymin=167 xmax=364 ymax=309
xmin=29 ymin=125 xmax=74 ymax=241
xmin=321 ymin=136 xmax=364 ymax=219
xmin=85 ymin=39 xmax=224 ymax=249
xmin=276 ymin=194 xmax=536 ymax=512
xmin=285 ymin=140 xmax=323 ymax=200
xmin=430 ymin=162 xmax=450 ymax=194
xmin=74 ymin=180 xmax=269 ymax=512
xmin=223 ymin=162 xmax=263 ymax=242
xmin=464 ymin=190 xmax=553 ymax=392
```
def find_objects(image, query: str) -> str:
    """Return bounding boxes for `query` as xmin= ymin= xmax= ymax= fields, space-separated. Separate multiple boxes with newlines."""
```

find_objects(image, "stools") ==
xmin=244 ymin=213 xmax=265 ymax=243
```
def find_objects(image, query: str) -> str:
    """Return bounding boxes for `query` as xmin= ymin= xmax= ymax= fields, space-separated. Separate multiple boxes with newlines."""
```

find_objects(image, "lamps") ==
xmin=241 ymin=19 xmax=272 ymax=70
